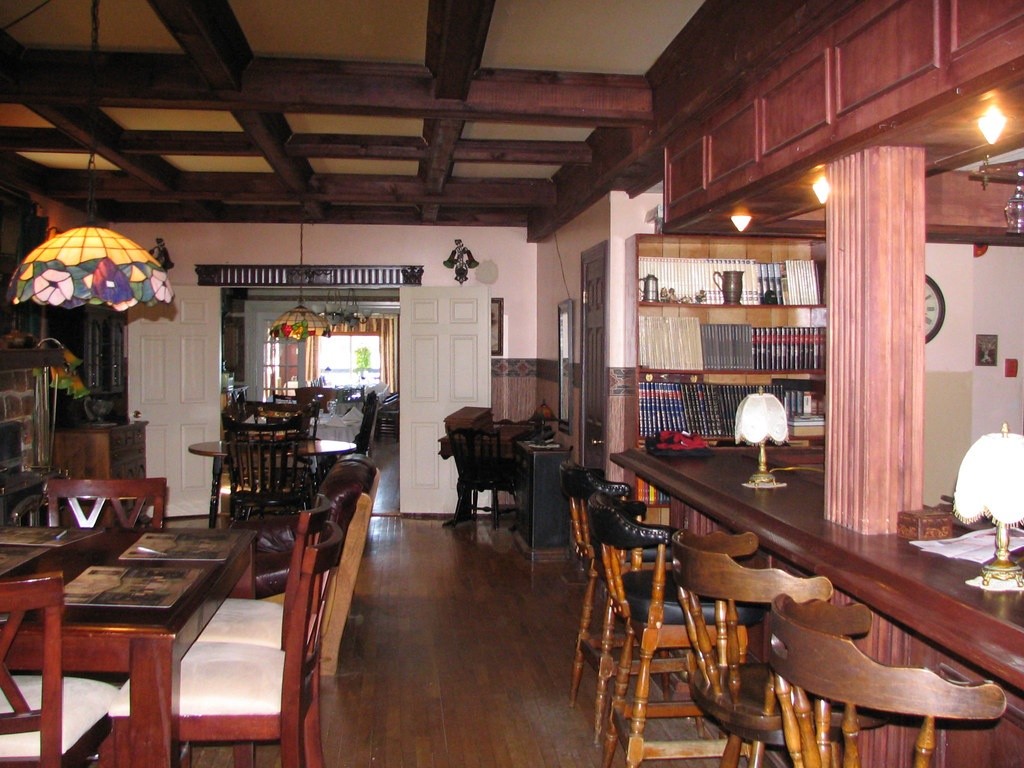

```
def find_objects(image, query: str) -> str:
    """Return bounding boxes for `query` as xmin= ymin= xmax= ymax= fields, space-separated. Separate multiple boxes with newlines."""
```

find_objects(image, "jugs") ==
xmin=638 ymin=275 xmax=659 ymax=303
xmin=713 ymin=271 xmax=744 ymax=306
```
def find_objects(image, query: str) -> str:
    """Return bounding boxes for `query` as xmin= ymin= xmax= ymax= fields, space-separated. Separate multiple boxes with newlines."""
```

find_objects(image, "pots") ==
xmin=4 ymin=330 xmax=36 ymax=348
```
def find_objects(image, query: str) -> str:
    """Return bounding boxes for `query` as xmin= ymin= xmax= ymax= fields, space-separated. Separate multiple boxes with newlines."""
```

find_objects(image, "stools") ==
xmin=559 ymin=454 xmax=1007 ymax=768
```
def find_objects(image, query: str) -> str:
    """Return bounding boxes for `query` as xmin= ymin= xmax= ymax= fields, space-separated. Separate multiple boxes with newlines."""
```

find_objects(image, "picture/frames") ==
xmin=558 ymin=298 xmax=572 ymax=436
xmin=491 ymin=298 xmax=503 ymax=356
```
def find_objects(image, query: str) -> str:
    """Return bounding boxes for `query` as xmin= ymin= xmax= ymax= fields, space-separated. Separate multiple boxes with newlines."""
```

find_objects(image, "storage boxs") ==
xmin=444 ymin=407 xmax=494 ymax=437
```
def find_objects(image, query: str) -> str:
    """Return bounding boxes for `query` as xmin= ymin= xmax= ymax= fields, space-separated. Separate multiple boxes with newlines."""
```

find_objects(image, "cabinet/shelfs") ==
xmin=625 ymin=234 xmax=828 ymax=506
xmin=56 ymin=418 xmax=147 ymax=523
xmin=515 ymin=441 xmax=569 ymax=561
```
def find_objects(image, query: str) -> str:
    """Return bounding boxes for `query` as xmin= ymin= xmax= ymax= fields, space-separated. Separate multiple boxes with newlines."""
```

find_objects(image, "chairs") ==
xmin=0 ymin=374 xmax=516 ymax=768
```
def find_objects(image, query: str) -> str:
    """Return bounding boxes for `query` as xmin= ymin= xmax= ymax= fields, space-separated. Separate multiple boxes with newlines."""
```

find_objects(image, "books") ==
xmin=636 ymin=254 xmax=827 ymax=437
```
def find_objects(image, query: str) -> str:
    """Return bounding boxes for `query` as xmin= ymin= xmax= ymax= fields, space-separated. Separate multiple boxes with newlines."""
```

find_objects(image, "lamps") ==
xmin=31 ymin=336 xmax=91 ymax=400
xmin=6 ymin=0 xmax=176 ymax=313
xmin=317 ymin=289 xmax=372 ymax=328
xmin=735 ymin=385 xmax=789 ymax=489
xmin=952 ymin=422 xmax=1024 ymax=592
xmin=526 ymin=400 xmax=561 ymax=448
xmin=269 ymin=149 xmax=332 ymax=340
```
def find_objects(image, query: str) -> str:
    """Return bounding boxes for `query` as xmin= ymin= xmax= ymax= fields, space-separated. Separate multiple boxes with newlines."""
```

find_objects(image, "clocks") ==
xmin=924 ymin=273 xmax=945 ymax=344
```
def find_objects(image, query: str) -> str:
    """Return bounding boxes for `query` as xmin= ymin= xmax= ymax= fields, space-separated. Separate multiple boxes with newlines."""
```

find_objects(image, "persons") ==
xmin=318 ymin=366 xmax=332 ymax=413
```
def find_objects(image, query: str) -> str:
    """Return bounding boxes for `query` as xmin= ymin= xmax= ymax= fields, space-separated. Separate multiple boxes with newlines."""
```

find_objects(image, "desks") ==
xmin=310 ymin=383 xmax=364 ymax=409
xmin=437 ymin=419 xmax=543 ymax=530
xmin=244 ymin=412 xmax=363 ymax=443
xmin=189 ymin=441 xmax=358 ymax=527
xmin=0 ymin=526 xmax=261 ymax=768
